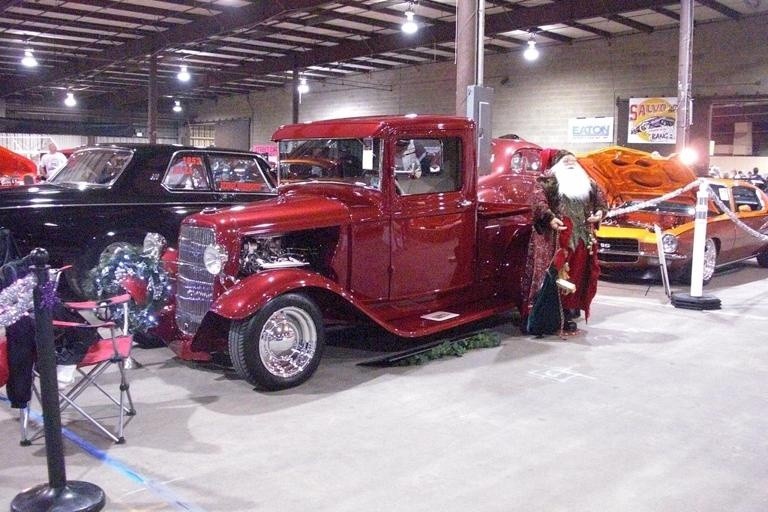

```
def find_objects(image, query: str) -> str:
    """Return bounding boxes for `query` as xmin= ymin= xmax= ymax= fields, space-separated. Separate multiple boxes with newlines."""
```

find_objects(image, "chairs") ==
xmin=3 ymin=254 xmax=138 ymax=447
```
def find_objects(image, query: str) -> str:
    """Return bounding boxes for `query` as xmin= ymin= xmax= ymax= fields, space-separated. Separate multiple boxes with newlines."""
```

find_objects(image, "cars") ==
xmin=574 ymin=145 xmax=768 ymax=286
xmin=142 ymin=111 xmax=605 ymax=392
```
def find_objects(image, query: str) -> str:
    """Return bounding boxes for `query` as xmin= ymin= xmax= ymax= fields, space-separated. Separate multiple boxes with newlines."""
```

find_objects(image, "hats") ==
xmin=541 ymin=148 xmax=575 ymax=177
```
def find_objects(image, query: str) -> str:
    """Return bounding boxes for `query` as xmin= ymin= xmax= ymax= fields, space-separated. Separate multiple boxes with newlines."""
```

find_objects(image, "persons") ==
xmin=722 ymin=172 xmax=730 ymax=179
xmin=747 ymin=171 xmax=753 ymax=180
xmin=728 ymin=169 xmax=737 ymax=180
xmin=38 ymin=142 xmax=69 ymax=181
xmin=736 ymin=171 xmax=747 ymax=179
xmin=750 ymin=167 xmax=765 ymax=181
xmin=516 ymin=147 xmax=608 ymax=342
xmin=395 ymin=138 xmax=431 ymax=178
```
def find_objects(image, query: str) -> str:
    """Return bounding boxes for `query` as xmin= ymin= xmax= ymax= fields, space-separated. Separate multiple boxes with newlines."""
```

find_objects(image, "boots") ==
xmin=562 ymin=309 xmax=576 ymax=332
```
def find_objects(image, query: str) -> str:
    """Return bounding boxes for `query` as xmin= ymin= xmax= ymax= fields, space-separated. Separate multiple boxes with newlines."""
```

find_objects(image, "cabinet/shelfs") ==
xmin=704 ymin=99 xmax=768 ymax=160
